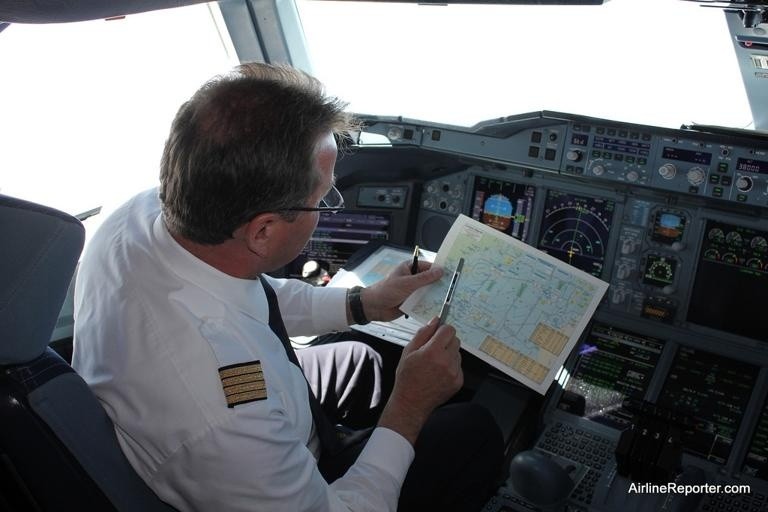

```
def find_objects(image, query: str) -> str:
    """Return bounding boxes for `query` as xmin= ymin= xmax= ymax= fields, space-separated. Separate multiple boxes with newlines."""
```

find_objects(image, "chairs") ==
xmin=0 ymin=194 xmax=183 ymax=510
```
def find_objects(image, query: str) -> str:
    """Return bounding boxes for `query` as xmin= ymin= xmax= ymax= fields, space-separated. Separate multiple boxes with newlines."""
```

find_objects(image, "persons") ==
xmin=71 ymin=63 xmax=464 ymax=510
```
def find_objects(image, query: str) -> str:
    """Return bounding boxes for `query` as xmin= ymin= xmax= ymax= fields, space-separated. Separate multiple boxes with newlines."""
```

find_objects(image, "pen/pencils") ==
xmin=439 ymin=258 xmax=464 ymax=326
xmin=405 ymin=245 xmax=418 ymax=318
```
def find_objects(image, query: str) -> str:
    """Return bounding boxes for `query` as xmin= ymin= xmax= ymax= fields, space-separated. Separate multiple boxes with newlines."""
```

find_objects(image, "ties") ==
xmin=258 ymin=273 xmax=334 ymax=487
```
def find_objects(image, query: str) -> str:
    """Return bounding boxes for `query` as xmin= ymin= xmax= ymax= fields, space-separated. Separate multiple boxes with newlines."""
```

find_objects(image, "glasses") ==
xmin=245 ymin=184 xmax=345 ymax=220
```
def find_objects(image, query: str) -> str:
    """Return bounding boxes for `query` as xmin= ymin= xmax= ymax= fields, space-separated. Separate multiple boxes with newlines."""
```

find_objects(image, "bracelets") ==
xmin=347 ymin=285 xmax=371 ymax=326
